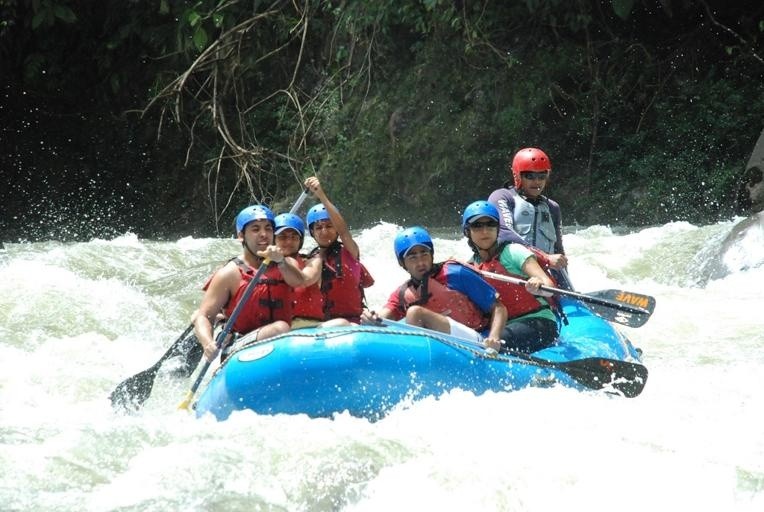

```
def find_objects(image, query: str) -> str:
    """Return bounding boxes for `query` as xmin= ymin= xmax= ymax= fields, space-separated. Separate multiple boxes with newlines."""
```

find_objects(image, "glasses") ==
xmin=469 ymin=221 xmax=498 ymax=228
xmin=522 ymin=171 xmax=547 ymax=180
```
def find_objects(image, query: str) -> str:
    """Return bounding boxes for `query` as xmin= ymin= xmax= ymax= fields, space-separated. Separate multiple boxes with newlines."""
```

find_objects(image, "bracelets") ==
xmin=277 ymin=256 xmax=285 ymax=266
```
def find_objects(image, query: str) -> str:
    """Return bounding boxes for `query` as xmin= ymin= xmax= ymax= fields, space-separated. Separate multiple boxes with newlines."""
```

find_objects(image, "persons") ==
xmin=190 ymin=213 xmax=323 ymax=330
xmin=462 ymin=201 xmax=561 ymax=353
xmin=305 ymin=176 xmax=374 ymax=324
xmin=194 ymin=205 xmax=304 ymax=364
xmin=488 ymin=147 xmax=568 ymax=287
xmin=359 ymin=226 xmax=508 ymax=354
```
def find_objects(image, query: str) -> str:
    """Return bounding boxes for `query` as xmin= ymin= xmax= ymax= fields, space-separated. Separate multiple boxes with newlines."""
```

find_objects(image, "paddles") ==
xmin=109 ymin=256 xmax=272 ymax=417
xmin=370 ymin=311 xmax=648 ymax=399
xmin=450 ymin=256 xmax=655 ymax=328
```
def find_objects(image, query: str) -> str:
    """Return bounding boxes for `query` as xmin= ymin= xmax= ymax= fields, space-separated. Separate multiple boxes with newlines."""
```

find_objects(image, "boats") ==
xmin=185 ymin=294 xmax=651 ymax=424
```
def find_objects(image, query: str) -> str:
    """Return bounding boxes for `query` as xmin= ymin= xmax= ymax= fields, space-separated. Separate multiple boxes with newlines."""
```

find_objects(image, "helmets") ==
xmin=236 ymin=201 xmax=330 ymax=250
xmin=462 ymin=200 xmax=499 ymax=233
xmin=512 ymin=148 xmax=552 ymax=190
xmin=394 ymin=227 xmax=434 ymax=261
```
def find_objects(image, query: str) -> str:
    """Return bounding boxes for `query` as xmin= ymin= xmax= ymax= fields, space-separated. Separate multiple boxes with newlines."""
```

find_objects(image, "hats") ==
xmin=468 ymin=215 xmax=498 ymax=223
xmin=403 ymin=243 xmax=431 ymax=258
